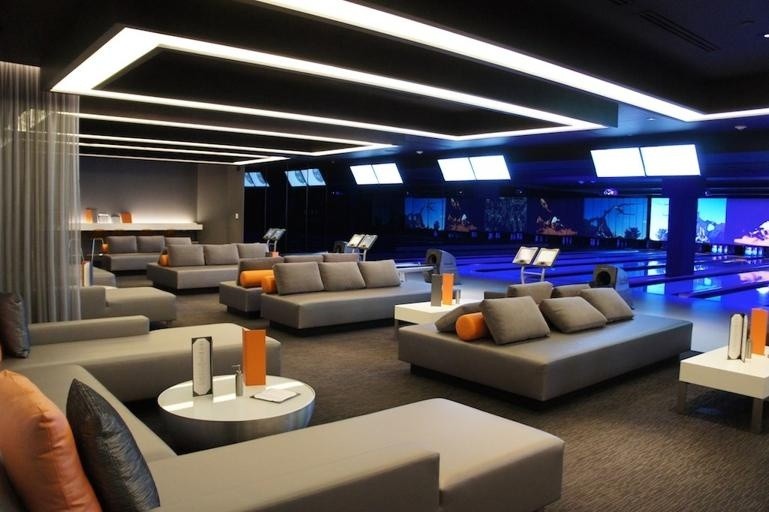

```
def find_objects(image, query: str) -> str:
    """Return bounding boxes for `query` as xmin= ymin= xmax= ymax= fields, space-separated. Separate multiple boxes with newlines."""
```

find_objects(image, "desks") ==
xmin=674 ymin=343 xmax=769 ymax=433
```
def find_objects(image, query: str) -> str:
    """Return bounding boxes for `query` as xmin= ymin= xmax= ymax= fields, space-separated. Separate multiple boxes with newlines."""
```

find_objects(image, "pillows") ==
xmin=108 ymin=233 xmax=271 ymax=265
